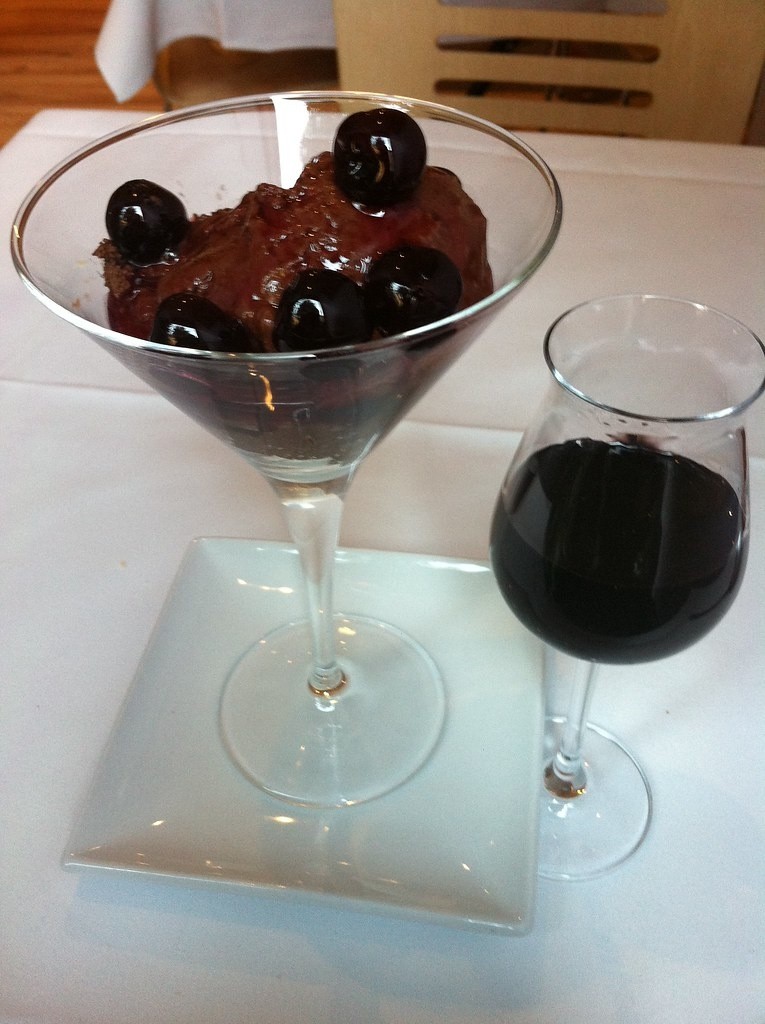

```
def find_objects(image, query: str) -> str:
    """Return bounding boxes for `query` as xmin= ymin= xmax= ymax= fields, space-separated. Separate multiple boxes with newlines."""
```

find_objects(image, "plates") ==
xmin=58 ymin=535 xmax=548 ymax=938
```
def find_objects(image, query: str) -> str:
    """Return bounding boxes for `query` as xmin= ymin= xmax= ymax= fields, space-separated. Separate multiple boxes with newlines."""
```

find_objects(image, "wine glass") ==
xmin=10 ymin=90 xmax=564 ymax=809
xmin=488 ymin=293 xmax=765 ymax=880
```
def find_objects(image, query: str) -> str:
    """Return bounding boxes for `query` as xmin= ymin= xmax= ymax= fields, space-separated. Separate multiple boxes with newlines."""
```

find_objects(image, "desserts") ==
xmin=94 ymin=106 xmax=493 ymax=498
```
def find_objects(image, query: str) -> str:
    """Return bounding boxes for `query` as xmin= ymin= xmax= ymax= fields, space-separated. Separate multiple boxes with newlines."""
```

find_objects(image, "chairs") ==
xmin=333 ymin=0 xmax=765 ymax=145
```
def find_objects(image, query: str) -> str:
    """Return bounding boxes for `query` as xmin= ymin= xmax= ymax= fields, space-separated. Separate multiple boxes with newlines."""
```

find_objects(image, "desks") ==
xmin=0 ymin=108 xmax=765 ymax=1024
xmin=92 ymin=0 xmax=670 ymax=107
